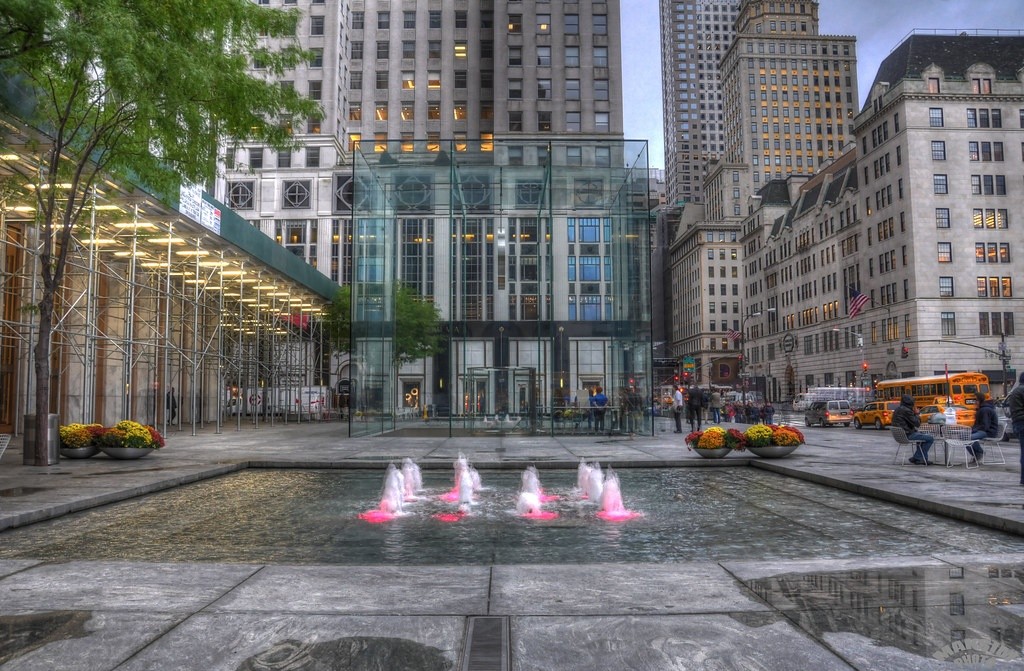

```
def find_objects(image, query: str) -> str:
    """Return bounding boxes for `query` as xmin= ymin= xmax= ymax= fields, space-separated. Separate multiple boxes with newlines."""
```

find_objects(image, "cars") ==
xmin=994 ymin=398 xmax=1015 ymax=440
xmin=918 ymin=399 xmax=977 ymax=429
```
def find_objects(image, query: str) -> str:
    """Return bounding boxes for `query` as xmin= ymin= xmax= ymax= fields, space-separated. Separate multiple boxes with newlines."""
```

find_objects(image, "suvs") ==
xmin=853 ymin=401 xmax=901 ymax=429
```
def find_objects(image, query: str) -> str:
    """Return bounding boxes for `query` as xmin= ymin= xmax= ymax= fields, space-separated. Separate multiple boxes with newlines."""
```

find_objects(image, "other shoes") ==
xmin=972 ymin=450 xmax=986 ymax=464
xmin=674 ymin=430 xmax=682 ymax=433
xmin=909 ymin=457 xmax=933 ymax=465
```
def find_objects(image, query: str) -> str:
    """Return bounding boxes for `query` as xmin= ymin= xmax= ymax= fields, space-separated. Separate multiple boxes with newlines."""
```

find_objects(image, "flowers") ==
xmin=59 ymin=421 xmax=165 ymax=450
xmin=684 ymin=423 xmax=804 ymax=459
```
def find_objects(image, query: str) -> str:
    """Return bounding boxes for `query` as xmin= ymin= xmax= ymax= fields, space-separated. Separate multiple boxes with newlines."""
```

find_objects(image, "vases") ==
xmin=694 ymin=447 xmax=732 ymax=459
xmin=746 ymin=445 xmax=799 ymax=458
xmin=101 ymin=448 xmax=154 ymax=460
xmin=59 ymin=445 xmax=101 ymax=459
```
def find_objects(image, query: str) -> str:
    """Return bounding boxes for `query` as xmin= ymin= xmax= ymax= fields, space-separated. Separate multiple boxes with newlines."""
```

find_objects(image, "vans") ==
xmin=805 ymin=400 xmax=854 ymax=428
xmin=792 ymin=393 xmax=816 ymax=411
xmin=721 ymin=393 xmax=754 ymax=403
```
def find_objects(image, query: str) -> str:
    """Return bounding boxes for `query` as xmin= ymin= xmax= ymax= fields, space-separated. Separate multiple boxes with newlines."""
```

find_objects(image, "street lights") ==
xmin=741 ymin=308 xmax=776 ymax=423
xmin=833 ymin=328 xmax=865 ymax=386
xmin=1000 ymin=329 xmax=1007 ymax=397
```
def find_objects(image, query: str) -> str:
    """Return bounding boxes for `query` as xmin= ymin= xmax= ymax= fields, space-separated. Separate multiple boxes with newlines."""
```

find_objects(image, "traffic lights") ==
xmin=739 ymin=355 xmax=748 ymax=366
xmin=674 ymin=373 xmax=678 ymax=380
xmin=901 ymin=347 xmax=909 ymax=358
xmin=683 ymin=372 xmax=687 ymax=381
xmin=863 ymin=363 xmax=867 ymax=376
xmin=680 ymin=388 xmax=686 ymax=392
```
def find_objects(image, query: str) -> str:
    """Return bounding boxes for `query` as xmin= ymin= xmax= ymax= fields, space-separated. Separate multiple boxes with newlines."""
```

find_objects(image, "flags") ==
xmin=727 ymin=328 xmax=741 ymax=343
xmin=848 ymin=287 xmax=869 ymax=319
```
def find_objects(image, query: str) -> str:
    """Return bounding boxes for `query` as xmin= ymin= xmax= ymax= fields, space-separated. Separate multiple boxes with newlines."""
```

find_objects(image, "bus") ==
xmin=691 ymin=385 xmax=732 ymax=395
xmin=874 ymin=373 xmax=991 ymax=409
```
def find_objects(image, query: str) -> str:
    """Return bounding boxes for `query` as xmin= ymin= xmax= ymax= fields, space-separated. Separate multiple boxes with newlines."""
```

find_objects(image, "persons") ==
xmin=688 ymin=383 xmax=710 ymax=432
xmin=888 ymin=393 xmax=934 ymax=465
xmin=709 ymin=388 xmax=722 ymax=425
xmin=1008 ymin=371 xmax=1024 ymax=485
xmin=964 ymin=392 xmax=999 ymax=465
xmin=722 ymin=402 xmax=776 ymax=426
xmin=166 ymin=387 xmax=178 ymax=425
xmin=590 ymin=386 xmax=608 ymax=431
xmin=627 ymin=385 xmax=644 ymax=433
xmin=671 ymin=384 xmax=684 ymax=433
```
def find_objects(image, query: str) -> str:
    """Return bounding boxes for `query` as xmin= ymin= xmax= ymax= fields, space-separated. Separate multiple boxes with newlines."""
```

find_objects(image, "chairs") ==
xmin=888 ymin=422 xmax=1009 ymax=470
xmin=391 ymin=405 xmax=421 ymax=422
